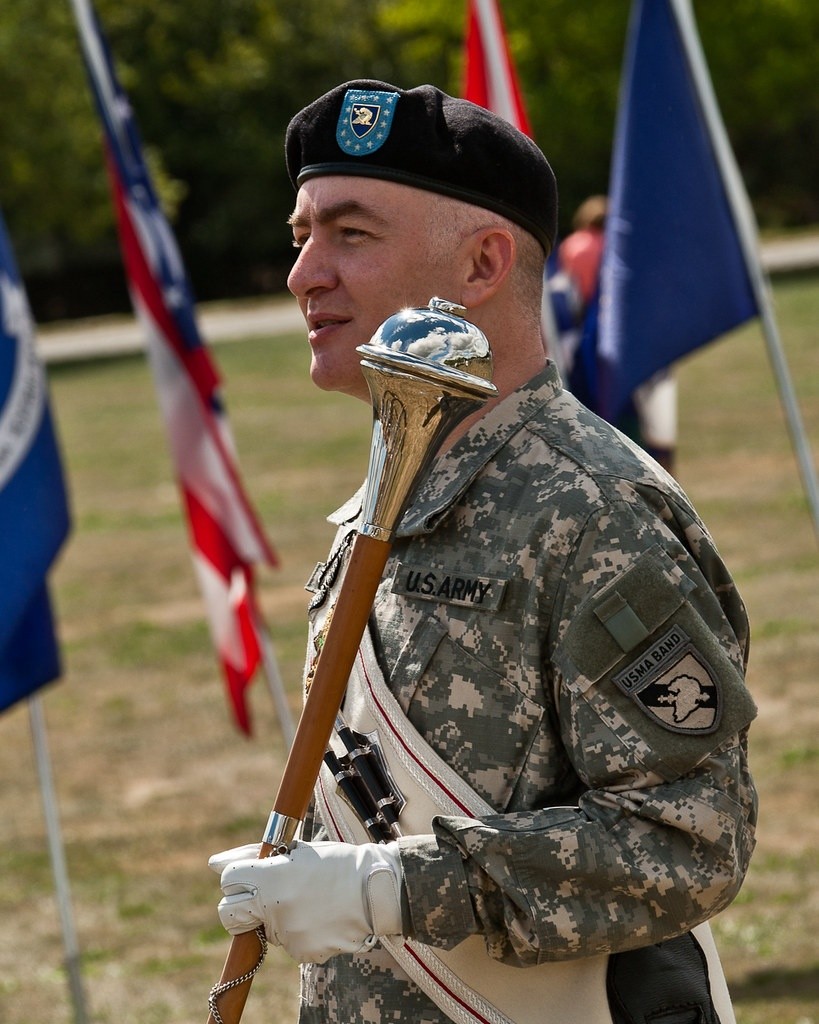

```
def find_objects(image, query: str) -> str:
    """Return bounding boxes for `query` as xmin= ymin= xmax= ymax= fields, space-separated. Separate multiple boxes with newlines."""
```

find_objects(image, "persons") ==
xmin=202 ymin=79 xmax=765 ymax=1024
xmin=539 ymin=194 xmax=682 ymax=473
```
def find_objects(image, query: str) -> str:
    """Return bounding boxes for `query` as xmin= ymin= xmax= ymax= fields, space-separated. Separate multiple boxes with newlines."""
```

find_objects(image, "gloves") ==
xmin=208 ymin=840 xmax=407 ymax=965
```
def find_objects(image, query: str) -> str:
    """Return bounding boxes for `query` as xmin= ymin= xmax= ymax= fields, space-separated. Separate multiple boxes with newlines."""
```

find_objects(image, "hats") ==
xmin=284 ymin=79 xmax=560 ymax=259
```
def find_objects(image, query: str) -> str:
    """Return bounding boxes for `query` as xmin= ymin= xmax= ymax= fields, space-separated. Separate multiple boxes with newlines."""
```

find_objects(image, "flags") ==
xmin=2 ymin=221 xmax=68 ymax=712
xmin=462 ymin=0 xmax=528 ymax=140
xmin=74 ymin=1 xmax=282 ymax=736
xmin=594 ymin=0 xmax=765 ymax=412
